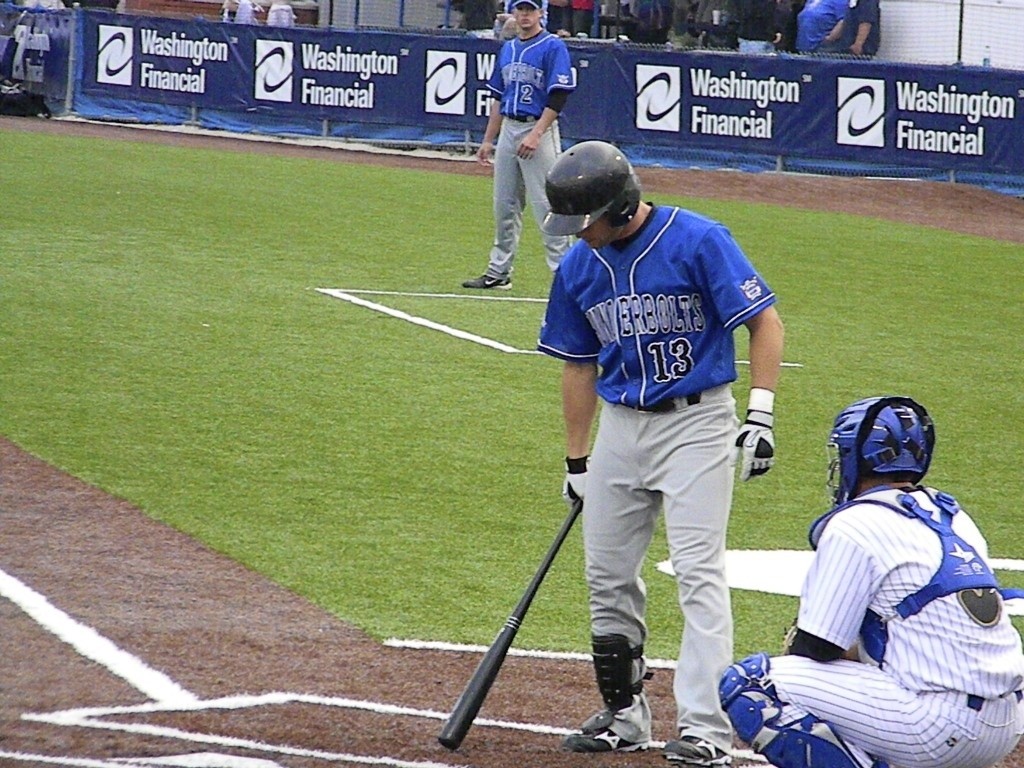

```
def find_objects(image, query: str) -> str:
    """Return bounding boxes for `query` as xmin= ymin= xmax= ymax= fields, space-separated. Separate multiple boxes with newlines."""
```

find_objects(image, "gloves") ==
xmin=562 ymin=455 xmax=591 ymax=513
xmin=728 ymin=409 xmax=775 ymax=482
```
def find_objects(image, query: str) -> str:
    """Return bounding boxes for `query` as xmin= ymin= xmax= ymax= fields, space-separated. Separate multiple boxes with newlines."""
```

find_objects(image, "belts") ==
xmin=509 ymin=115 xmax=541 ymax=123
xmin=967 ymin=690 xmax=1023 ymax=711
xmin=619 ymin=391 xmax=702 ymax=413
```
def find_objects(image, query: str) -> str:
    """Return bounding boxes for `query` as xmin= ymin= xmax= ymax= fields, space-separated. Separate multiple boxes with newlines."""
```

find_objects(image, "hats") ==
xmin=512 ymin=0 xmax=542 ymax=9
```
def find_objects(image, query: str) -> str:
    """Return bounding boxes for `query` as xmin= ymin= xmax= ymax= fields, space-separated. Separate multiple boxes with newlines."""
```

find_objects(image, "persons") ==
xmin=718 ymin=396 xmax=1024 ymax=768
xmin=493 ymin=0 xmax=881 ymax=61
xmin=462 ymin=0 xmax=576 ymax=291
xmin=537 ymin=141 xmax=786 ymax=768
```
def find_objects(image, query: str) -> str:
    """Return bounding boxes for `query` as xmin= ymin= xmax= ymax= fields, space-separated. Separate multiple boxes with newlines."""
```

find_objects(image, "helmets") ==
xmin=823 ymin=396 xmax=936 ymax=509
xmin=537 ymin=140 xmax=641 ymax=237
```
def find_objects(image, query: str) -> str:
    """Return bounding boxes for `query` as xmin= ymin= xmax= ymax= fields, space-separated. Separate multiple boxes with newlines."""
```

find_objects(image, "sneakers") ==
xmin=565 ymin=725 xmax=650 ymax=753
xmin=462 ymin=274 xmax=512 ymax=290
xmin=663 ymin=735 xmax=732 ymax=766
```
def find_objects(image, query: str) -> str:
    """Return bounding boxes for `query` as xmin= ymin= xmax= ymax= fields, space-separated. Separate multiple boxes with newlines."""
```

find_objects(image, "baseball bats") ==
xmin=436 ymin=499 xmax=583 ymax=752
